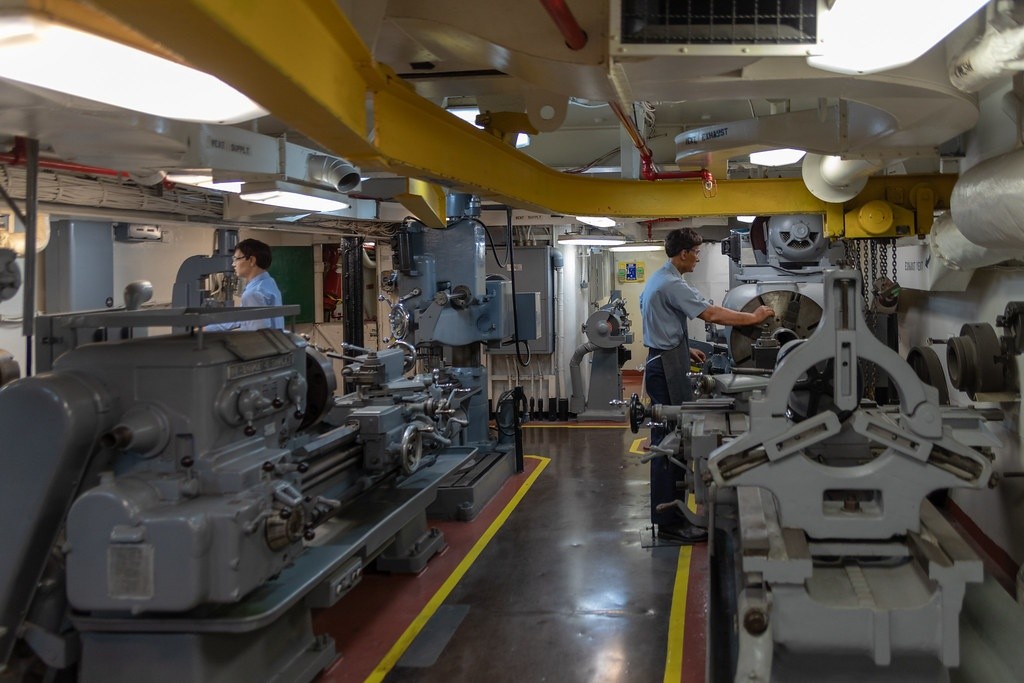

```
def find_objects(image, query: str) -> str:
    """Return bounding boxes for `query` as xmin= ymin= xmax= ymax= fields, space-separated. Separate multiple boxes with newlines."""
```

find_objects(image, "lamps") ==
xmin=1 ymin=5 xmax=271 ymax=125
xmin=609 ymin=243 xmax=665 ymax=252
xmin=440 ymin=96 xmax=531 ymax=148
xmin=557 ymin=235 xmax=627 ymax=246
xmin=238 ymin=180 xmax=351 ymax=212
xmin=576 ymin=215 xmax=616 ymax=228
xmin=166 ymin=169 xmax=249 ymax=193
xmin=805 ymin=0 xmax=990 ymax=75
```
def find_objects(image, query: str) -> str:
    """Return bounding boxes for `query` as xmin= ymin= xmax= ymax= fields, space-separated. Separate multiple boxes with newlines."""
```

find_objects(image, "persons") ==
xmin=638 ymin=227 xmax=776 ymax=541
xmin=194 ymin=238 xmax=284 ymax=331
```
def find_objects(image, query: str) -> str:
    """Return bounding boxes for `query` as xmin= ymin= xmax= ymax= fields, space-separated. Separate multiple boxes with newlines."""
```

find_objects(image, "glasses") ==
xmin=690 ymin=248 xmax=701 ymax=257
xmin=232 ymin=254 xmax=250 ymax=261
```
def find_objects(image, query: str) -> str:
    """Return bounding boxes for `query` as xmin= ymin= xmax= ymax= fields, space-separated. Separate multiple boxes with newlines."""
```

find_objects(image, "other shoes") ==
xmin=657 ymin=523 xmax=709 ymax=543
